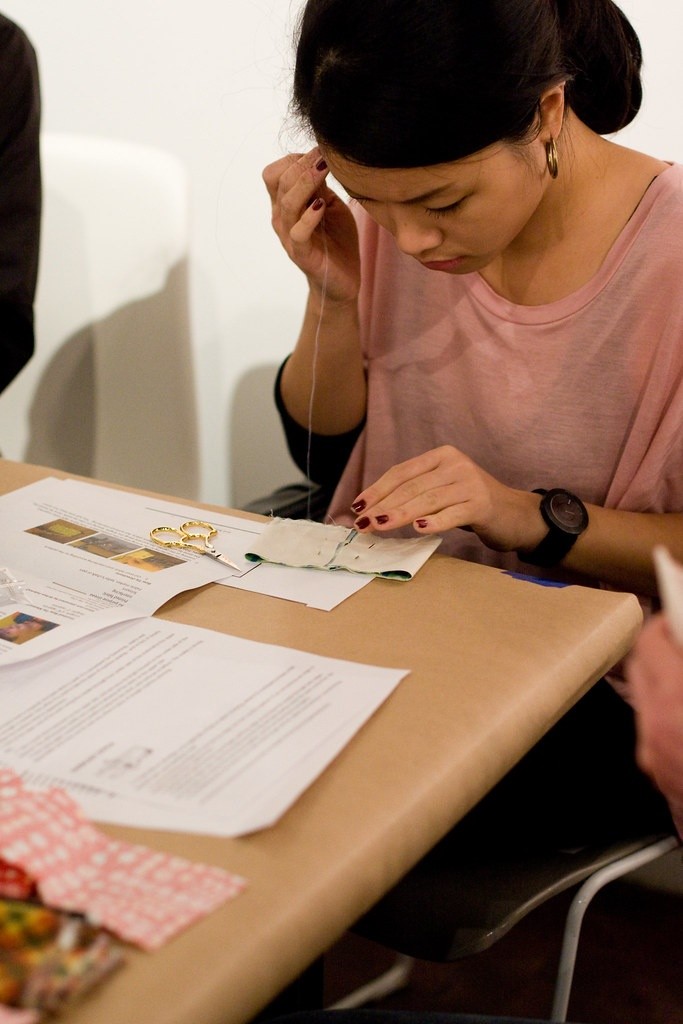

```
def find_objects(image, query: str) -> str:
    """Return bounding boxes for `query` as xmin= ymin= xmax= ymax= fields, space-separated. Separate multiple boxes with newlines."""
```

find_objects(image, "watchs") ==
xmin=517 ymin=488 xmax=589 ymax=567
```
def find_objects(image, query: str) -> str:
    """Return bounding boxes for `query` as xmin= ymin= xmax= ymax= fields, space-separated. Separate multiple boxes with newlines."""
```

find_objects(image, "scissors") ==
xmin=149 ymin=519 xmax=244 ymax=574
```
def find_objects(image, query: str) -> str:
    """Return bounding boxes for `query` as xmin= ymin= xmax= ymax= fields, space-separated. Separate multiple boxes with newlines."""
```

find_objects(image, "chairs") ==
xmin=235 ymin=479 xmax=683 ymax=1024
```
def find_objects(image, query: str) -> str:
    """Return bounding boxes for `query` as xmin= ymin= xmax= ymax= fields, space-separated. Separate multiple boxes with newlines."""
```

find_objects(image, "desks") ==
xmin=1 ymin=460 xmax=644 ymax=1024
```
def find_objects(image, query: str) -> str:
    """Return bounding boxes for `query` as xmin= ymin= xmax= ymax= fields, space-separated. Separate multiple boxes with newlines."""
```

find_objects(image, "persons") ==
xmin=0 ymin=614 xmax=58 ymax=641
xmin=248 ymin=0 xmax=683 ymax=1024
xmin=0 ymin=14 xmax=43 ymax=396
